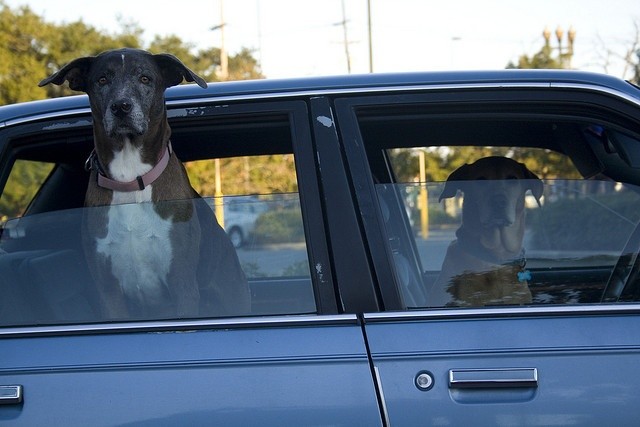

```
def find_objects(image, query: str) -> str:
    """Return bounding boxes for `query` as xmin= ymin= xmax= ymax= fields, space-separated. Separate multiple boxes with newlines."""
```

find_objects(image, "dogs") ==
xmin=424 ymin=156 xmax=547 ymax=307
xmin=37 ymin=47 xmax=251 ymax=321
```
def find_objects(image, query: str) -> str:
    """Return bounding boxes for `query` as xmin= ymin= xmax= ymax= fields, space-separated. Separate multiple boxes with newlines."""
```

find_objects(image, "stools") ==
xmin=0 ymin=248 xmax=112 ymax=324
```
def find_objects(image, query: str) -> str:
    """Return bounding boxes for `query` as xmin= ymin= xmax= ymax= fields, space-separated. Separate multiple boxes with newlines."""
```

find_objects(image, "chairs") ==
xmin=372 ymin=176 xmax=427 ymax=307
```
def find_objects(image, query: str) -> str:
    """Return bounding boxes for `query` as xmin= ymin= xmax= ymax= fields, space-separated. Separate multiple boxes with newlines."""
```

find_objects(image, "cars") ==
xmin=0 ymin=68 xmax=640 ymax=427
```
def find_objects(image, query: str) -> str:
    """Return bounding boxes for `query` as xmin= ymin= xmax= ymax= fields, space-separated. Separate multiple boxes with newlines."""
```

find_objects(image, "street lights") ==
xmin=542 ymin=23 xmax=575 ymax=69
